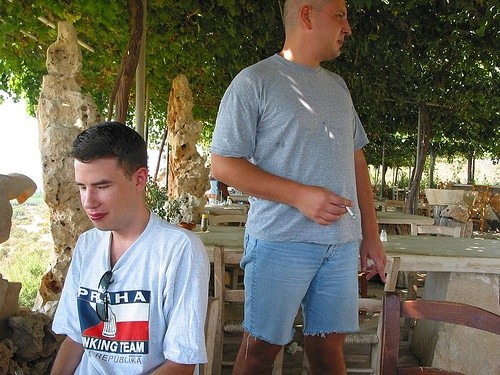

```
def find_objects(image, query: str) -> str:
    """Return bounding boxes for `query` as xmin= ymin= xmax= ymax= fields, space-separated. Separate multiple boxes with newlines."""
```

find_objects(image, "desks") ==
xmin=206 ymin=213 xmax=435 ymax=289
xmin=193 ymin=232 xmax=500 ymax=375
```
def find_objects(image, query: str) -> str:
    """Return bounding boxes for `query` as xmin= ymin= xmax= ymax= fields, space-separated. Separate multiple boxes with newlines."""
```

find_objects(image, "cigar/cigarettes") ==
xmin=211 ymin=0 xmax=387 ymax=375
xmin=346 ymin=206 xmax=356 ymax=220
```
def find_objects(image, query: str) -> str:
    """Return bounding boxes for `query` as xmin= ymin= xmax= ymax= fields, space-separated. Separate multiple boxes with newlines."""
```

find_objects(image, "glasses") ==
xmin=96 ymin=269 xmax=115 ymax=322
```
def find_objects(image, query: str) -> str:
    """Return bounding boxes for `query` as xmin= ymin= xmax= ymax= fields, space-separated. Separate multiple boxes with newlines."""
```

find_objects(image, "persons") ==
xmin=50 ymin=121 xmax=210 ymax=375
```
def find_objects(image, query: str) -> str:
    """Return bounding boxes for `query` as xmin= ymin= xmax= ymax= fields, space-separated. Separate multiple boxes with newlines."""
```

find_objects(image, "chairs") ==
xmin=300 ymin=256 xmax=400 ymax=375
xmin=206 ymin=245 xmax=285 ymax=375
xmin=380 ymin=291 xmax=500 ymax=375
xmin=403 ymin=222 xmax=461 ymax=342
xmin=417 ymin=188 xmax=478 ymax=239
